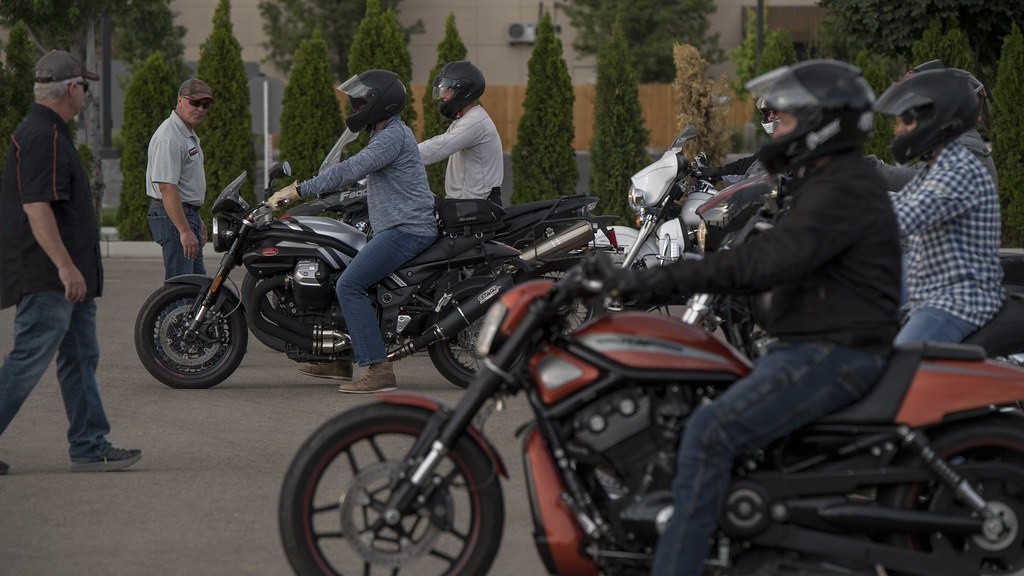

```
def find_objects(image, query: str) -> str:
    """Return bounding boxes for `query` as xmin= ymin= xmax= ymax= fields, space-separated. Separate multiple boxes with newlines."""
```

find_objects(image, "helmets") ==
xmin=757 ymin=59 xmax=877 ymax=190
xmin=432 ymin=61 xmax=485 ymax=120
xmin=345 ymin=69 xmax=408 ymax=133
xmin=887 ymin=59 xmax=987 ymax=165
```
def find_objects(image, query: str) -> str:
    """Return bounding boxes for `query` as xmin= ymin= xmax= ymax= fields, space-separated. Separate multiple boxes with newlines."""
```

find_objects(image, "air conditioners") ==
xmin=506 ymin=20 xmax=536 ymax=43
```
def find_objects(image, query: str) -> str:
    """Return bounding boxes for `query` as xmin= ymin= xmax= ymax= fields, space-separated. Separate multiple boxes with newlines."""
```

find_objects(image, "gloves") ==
xmin=582 ymin=251 xmax=681 ymax=306
xmin=268 ymin=180 xmax=302 ymax=211
xmin=702 ymin=166 xmax=720 ymax=186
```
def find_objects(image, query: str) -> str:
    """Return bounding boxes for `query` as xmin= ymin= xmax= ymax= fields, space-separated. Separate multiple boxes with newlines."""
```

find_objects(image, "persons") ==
xmin=1 ymin=50 xmax=144 ymax=476
xmin=355 ymin=59 xmax=507 ymax=208
xmin=267 ymin=69 xmax=440 ymax=391
xmin=605 ymin=59 xmax=1005 ymax=575
xmin=145 ymin=77 xmax=215 ymax=283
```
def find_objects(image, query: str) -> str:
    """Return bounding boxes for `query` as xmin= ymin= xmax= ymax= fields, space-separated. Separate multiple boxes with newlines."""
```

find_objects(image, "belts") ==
xmin=154 ymin=198 xmax=200 ymax=211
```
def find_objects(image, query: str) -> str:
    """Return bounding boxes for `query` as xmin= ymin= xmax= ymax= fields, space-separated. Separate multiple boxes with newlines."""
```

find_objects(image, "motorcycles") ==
xmin=574 ymin=126 xmax=774 ymax=313
xmin=134 ymin=168 xmax=544 ymax=390
xmin=679 ymin=170 xmax=1024 ymax=369
xmin=317 ymin=127 xmax=601 ymax=252
xmin=277 ymin=247 xmax=1024 ymax=576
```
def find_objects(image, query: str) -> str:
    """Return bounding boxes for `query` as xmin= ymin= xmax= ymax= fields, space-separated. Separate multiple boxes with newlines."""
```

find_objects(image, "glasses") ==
xmin=900 ymin=112 xmax=915 ymax=124
xmin=67 ymin=81 xmax=90 ymax=93
xmin=186 ymin=98 xmax=212 ymax=109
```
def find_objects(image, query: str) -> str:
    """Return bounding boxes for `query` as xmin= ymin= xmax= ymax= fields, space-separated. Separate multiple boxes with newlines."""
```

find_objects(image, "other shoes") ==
xmin=70 ymin=446 xmax=142 ymax=472
xmin=0 ymin=461 xmax=10 ymax=475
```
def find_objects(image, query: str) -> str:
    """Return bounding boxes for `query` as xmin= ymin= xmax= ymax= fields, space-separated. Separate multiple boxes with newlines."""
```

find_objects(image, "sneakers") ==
xmin=339 ymin=362 xmax=397 ymax=393
xmin=298 ymin=361 xmax=353 ymax=381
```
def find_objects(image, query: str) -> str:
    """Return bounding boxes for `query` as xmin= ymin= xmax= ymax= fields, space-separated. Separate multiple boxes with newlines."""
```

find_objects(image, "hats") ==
xmin=178 ymin=78 xmax=213 ymax=100
xmin=32 ymin=49 xmax=100 ymax=83
